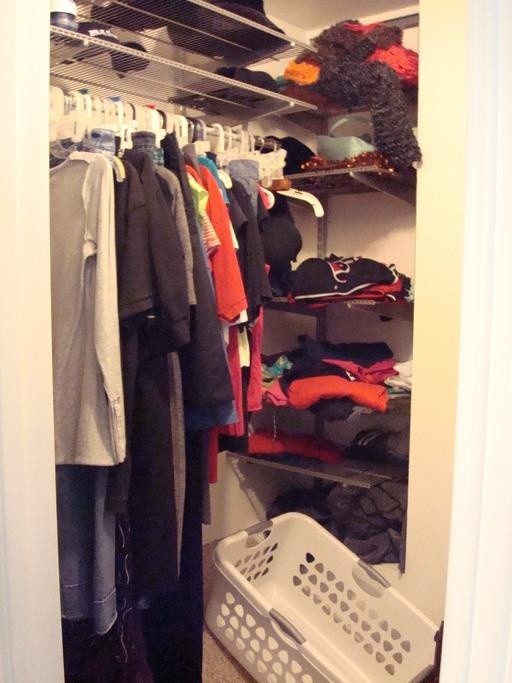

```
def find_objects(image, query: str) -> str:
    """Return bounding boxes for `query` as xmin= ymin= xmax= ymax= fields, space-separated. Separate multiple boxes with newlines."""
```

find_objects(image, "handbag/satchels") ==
xmin=316 ymin=115 xmax=374 ymax=162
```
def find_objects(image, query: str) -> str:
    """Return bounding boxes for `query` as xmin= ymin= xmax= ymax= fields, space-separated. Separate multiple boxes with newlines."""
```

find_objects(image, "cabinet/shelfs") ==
xmin=222 ymin=90 xmax=414 ymax=570
xmin=51 ymin=0 xmax=320 ymax=69
xmin=52 ymin=24 xmax=317 ymax=131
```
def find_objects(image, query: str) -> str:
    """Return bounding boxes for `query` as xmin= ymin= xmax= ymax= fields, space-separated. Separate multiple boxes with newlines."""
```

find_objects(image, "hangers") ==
xmin=50 ymin=87 xmax=328 ymax=221
